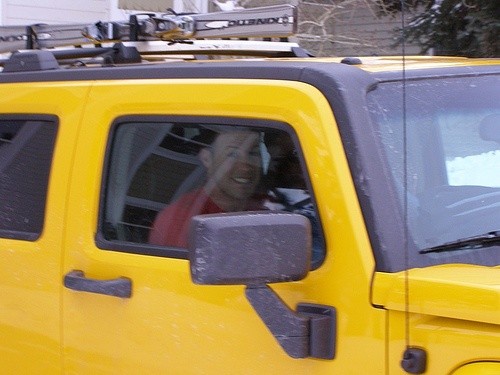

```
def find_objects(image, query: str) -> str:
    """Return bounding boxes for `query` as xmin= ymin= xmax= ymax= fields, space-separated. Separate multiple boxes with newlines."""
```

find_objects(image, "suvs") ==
xmin=0 ymin=4 xmax=500 ymax=375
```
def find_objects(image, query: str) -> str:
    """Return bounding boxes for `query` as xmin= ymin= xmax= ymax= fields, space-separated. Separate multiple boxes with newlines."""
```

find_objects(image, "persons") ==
xmin=265 ymin=130 xmax=327 ymax=266
xmin=144 ymin=124 xmax=274 ymax=255
